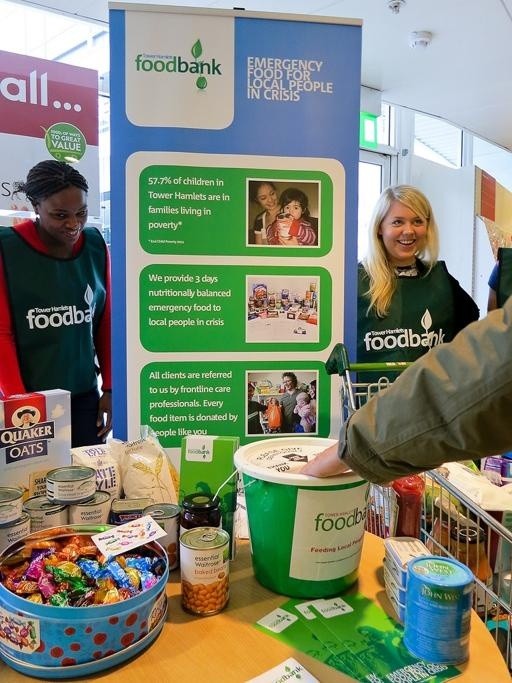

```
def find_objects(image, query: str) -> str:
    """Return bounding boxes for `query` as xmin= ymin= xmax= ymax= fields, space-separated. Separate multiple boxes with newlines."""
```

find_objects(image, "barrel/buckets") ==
xmin=235 ymin=436 xmax=369 ymax=599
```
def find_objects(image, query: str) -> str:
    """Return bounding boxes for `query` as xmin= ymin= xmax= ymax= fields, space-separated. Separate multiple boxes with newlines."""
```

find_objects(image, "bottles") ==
xmin=392 ymin=475 xmax=425 ymax=539
xmin=449 ymin=523 xmax=492 ymax=617
xmin=267 ymin=398 xmax=283 ymax=428
xmin=423 ymin=508 xmax=461 ymax=554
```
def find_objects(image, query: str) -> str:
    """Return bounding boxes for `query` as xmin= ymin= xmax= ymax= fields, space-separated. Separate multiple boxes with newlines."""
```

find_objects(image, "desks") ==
xmin=1 ymin=520 xmax=512 ymax=682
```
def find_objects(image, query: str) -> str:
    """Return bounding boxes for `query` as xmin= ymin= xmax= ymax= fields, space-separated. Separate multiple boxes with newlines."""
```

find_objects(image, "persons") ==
xmin=17 ymin=409 xmax=35 ymax=428
xmin=0 ymin=160 xmax=112 ymax=449
xmin=266 ymin=188 xmax=316 ymax=246
xmin=248 ymin=372 xmax=317 ymax=434
xmin=487 ymin=247 xmax=512 ymax=312
xmin=249 ymin=180 xmax=282 ymax=245
xmin=299 ymin=292 xmax=512 ymax=486
xmin=356 ymin=185 xmax=479 ymax=411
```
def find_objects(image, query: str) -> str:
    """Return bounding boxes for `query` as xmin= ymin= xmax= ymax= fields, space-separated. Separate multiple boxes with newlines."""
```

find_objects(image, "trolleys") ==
xmin=319 ymin=340 xmax=511 ymax=673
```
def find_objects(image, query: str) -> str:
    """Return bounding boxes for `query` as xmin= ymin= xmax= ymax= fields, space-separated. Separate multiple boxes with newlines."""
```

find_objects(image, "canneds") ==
xmin=0 ymin=465 xmax=231 ymax=616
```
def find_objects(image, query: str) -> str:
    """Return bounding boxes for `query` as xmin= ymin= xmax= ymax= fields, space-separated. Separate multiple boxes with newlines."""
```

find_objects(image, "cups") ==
xmin=276 ymin=211 xmax=293 ymax=241
